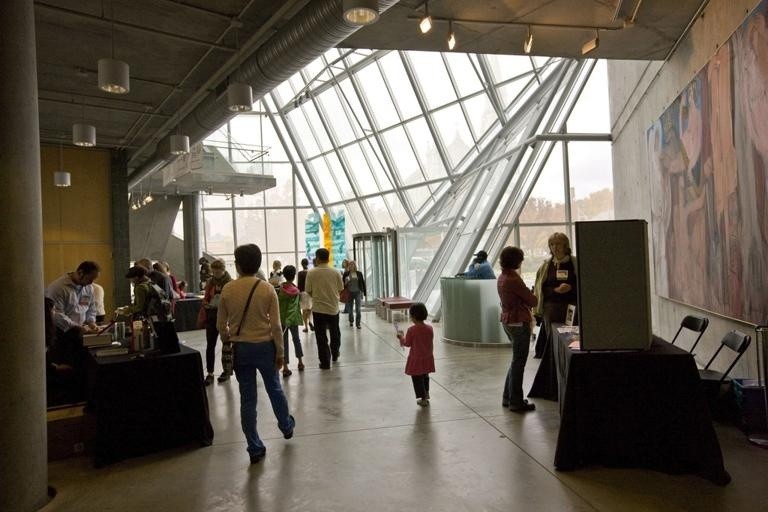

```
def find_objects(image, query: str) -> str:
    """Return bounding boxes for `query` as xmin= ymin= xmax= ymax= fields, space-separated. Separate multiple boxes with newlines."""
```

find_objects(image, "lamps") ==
xmin=671 ymin=316 xmax=709 ymax=353
xmin=697 ymin=329 xmax=751 ymax=411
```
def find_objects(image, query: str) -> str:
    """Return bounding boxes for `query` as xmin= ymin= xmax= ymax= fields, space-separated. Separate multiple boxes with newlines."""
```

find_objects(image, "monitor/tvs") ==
xmin=81 ymin=321 xmax=151 ymax=357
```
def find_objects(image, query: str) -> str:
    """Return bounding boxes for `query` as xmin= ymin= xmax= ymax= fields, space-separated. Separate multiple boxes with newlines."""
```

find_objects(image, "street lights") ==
xmin=196 ymin=293 xmax=211 ymax=329
xmin=340 ymin=288 xmax=352 ymax=303
xmin=222 ymin=343 xmax=234 ymax=376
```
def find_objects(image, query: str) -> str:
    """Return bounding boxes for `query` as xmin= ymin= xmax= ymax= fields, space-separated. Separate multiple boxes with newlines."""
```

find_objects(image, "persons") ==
xmin=532 ymin=232 xmax=579 ymax=357
xmin=198 ymin=251 xmax=234 ymax=386
xmin=216 ymin=243 xmax=295 ymax=464
xmin=497 ymin=246 xmax=538 ymax=414
xmin=270 ymin=248 xmax=367 ymax=377
xmin=400 ymin=302 xmax=436 ymax=406
xmin=255 ymin=267 xmax=267 ymax=282
xmin=123 ymin=258 xmax=188 ymax=322
xmin=464 ymin=251 xmax=497 ymax=279
xmin=45 ymin=260 xmax=105 ymax=346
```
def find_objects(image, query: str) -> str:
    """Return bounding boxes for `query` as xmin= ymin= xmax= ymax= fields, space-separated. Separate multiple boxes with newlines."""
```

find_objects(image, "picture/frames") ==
xmin=84 ymin=344 xmax=214 ymax=469
xmin=175 ymin=297 xmax=205 ymax=332
xmin=527 ymin=322 xmax=732 ymax=487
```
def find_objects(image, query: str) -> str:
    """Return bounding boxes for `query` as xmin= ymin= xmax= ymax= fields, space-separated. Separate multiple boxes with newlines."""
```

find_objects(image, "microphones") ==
xmin=385 ymin=300 xmax=417 ymax=324
xmin=376 ymin=297 xmax=410 ymax=320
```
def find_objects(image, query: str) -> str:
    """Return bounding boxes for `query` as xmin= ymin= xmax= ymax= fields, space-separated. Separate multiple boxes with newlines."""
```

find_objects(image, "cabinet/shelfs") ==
xmin=130 ymin=311 xmax=145 ymax=352
xmin=133 ymin=317 xmax=143 ymax=351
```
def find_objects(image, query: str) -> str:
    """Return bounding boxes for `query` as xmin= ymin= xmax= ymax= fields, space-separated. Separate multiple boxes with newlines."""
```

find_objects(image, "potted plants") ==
xmin=648 ymin=0 xmax=768 ymax=328
xmin=565 ymin=304 xmax=577 ymax=326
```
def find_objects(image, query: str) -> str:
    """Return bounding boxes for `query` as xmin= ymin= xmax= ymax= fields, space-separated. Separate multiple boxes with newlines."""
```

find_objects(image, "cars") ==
xmin=115 ymin=322 xmax=125 ymax=340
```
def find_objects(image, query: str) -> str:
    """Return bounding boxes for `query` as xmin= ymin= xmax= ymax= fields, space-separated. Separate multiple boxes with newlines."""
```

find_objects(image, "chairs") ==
xmin=319 ymin=364 xmax=330 ymax=369
xmin=510 ymin=403 xmax=534 ymax=411
xmin=417 ymin=400 xmax=428 ymax=406
xmin=284 ymin=415 xmax=295 ymax=439
xmin=250 ymin=446 xmax=266 ymax=464
xmin=298 ymin=363 xmax=304 ymax=371
xmin=217 ymin=373 xmax=230 ymax=382
xmin=332 ymin=352 xmax=340 ymax=361
xmin=204 ymin=375 xmax=214 ymax=386
xmin=283 ymin=369 xmax=291 ymax=377
xmin=503 ymin=400 xmax=528 ymax=406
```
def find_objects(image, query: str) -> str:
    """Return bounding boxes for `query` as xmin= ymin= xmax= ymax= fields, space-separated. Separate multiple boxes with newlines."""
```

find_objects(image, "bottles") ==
xmin=474 ymin=251 xmax=487 ymax=257
xmin=125 ymin=266 xmax=147 ymax=278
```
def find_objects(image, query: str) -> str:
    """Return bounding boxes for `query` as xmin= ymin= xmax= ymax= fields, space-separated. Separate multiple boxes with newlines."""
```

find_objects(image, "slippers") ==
xmin=143 ymin=281 xmax=167 ymax=318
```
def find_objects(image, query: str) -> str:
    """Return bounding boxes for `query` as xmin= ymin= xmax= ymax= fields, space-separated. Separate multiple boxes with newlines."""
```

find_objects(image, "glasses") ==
xmin=72 ymin=94 xmax=96 ymax=147
xmin=169 ymin=88 xmax=191 ymax=155
xmin=524 ymin=24 xmax=533 ymax=53
xmin=131 ymin=176 xmax=153 ymax=210
xmin=54 ymin=144 xmax=71 ymax=187
xmin=342 ymin=0 xmax=379 ymax=25
xmin=419 ymin=0 xmax=432 ymax=34
xmin=447 ymin=20 xmax=456 ymax=50
xmin=227 ymin=21 xmax=253 ymax=114
xmin=97 ymin=0 xmax=130 ymax=95
